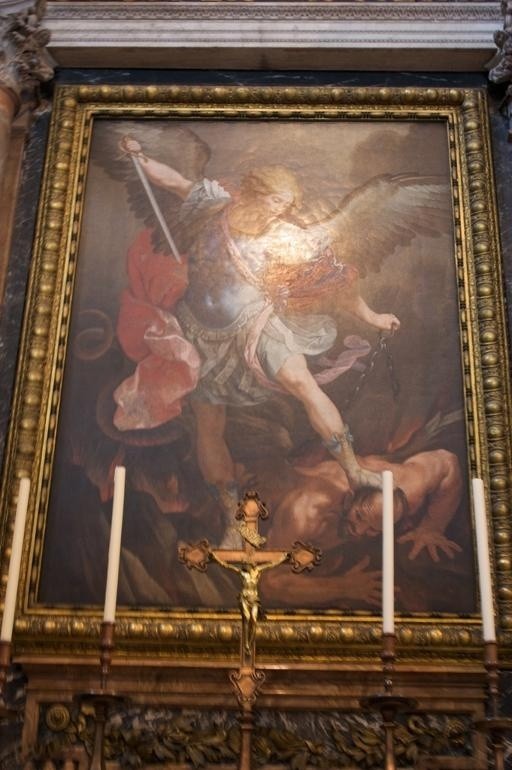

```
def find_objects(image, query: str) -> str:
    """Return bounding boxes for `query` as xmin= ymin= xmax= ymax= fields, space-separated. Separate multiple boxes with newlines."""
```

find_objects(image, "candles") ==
xmin=1 ymin=478 xmax=31 ymax=643
xmin=472 ymin=478 xmax=497 ymax=643
xmin=102 ymin=467 xmax=127 ymax=623
xmin=381 ymin=471 xmax=396 ymax=635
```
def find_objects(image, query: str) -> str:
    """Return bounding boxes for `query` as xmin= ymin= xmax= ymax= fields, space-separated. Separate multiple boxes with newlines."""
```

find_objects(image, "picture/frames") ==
xmin=0 ymin=81 xmax=512 ymax=668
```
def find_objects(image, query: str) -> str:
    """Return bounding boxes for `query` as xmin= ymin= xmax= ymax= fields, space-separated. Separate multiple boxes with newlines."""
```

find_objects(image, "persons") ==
xmin=119 ymin=140 xmax=398 ymax=552
xmin=216 ymin=557 xmax=285 ymax=659
xmin=262 ymin=442 xmax=461 ymax=606
